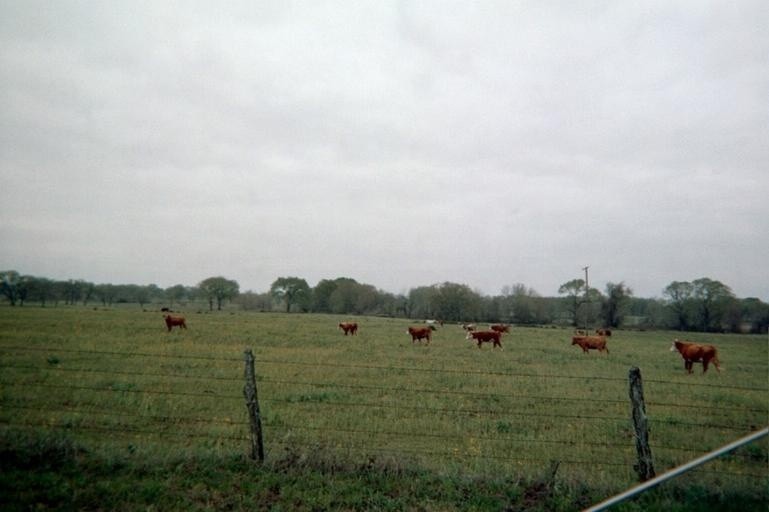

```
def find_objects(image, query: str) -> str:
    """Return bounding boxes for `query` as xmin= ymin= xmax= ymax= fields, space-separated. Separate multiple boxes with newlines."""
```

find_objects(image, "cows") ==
xmin=337 ymin=323 xmax=358 ymax=336
xmin=406 ymin=327 xmax=432 ymax=343
xmin=570 ymin=327 xmax=612 ymax=354
xmin=668 ymin=338 xmax=723 ymax=373
xmin=162 ymin=314 xmax=188 ymax=330
xmin=462 ymin=323 xmax=509 ymax=350
